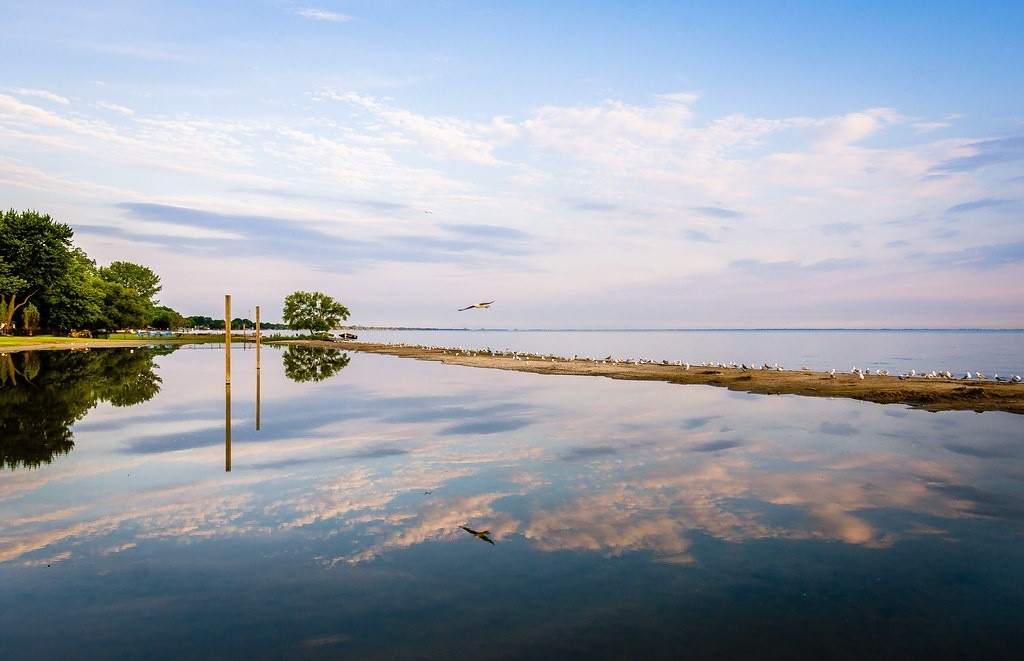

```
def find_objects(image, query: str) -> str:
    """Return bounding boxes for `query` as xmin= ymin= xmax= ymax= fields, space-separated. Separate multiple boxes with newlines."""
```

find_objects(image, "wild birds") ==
xmin=457 ymin=300 xmax=495 ymax=311
xmin=1010 ymin=374 xmax=1022 ymax=382
xmin=378 ymin=341 xmax=954 ymax=380
xmin=976 ymin=371 xmax=989 ymax=380
xmin=993 ymin=373 xmax=1006 ymax=381
xmin=458 ymin=526 xmax=496 ymax=547
xmin=961 ymin=371 xmax=972 ymax=380
